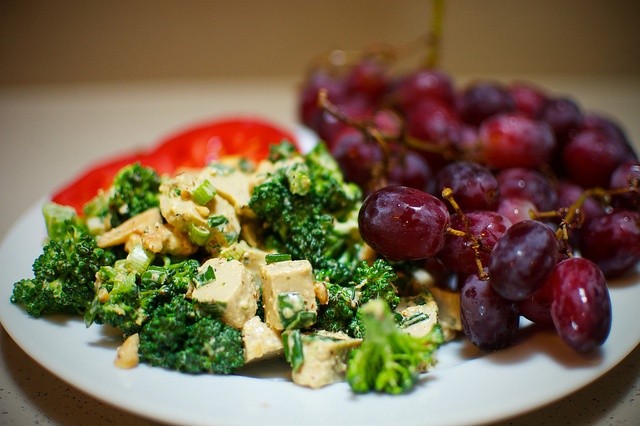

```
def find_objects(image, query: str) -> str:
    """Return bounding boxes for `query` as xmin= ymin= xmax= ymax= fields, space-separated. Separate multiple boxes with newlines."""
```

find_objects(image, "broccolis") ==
xmin=10 ymin=141 xmax=438 ymax=395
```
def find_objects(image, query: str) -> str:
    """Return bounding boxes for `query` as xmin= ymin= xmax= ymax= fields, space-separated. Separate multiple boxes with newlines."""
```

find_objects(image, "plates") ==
xmin=0 ymin=190 xmax=636 ymax=426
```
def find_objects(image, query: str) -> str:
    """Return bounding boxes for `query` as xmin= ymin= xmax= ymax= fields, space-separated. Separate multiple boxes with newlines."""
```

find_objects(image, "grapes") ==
xmin=303 ymin=54 xmax=640 ymax=354
